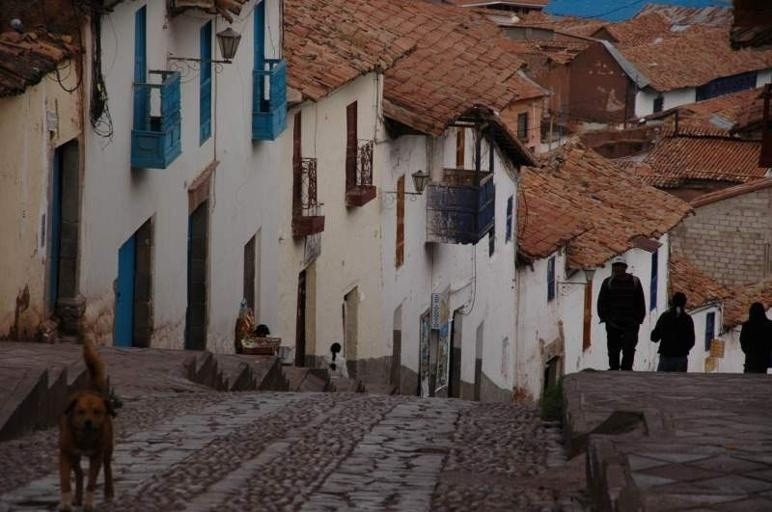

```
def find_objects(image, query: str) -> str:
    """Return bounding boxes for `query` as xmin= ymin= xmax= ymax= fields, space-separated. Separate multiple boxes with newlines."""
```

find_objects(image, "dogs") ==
xmin=58 ymin=335 xmax=124 ymax=512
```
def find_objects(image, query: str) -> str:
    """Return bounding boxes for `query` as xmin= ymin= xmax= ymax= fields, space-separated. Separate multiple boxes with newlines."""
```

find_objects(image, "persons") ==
xmin=254 ymin=322 xmax=277 ymax=356
xmin=595 ymin=256 xmax=646 ymax=372
xmin=318 ymin=342 xmax=350 ymax=379
xmin=649 ymin=291 xmax=695 ymax=372
xmin=738 ymin=301 xmax=771 ymax=375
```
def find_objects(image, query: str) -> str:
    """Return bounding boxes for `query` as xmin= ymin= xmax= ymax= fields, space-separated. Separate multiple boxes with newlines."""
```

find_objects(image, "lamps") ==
xmin=558 ymin=267 xmax=595 ymax=295
xmin=166 ymin=26 xmax=241 ymax=83
xmin=382 ymin=170 xmax=431 ymax=202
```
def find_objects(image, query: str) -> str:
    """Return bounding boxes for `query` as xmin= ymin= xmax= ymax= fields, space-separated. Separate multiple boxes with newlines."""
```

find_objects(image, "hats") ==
xmin=612 ymin=256 xmax=628 ymax=266
